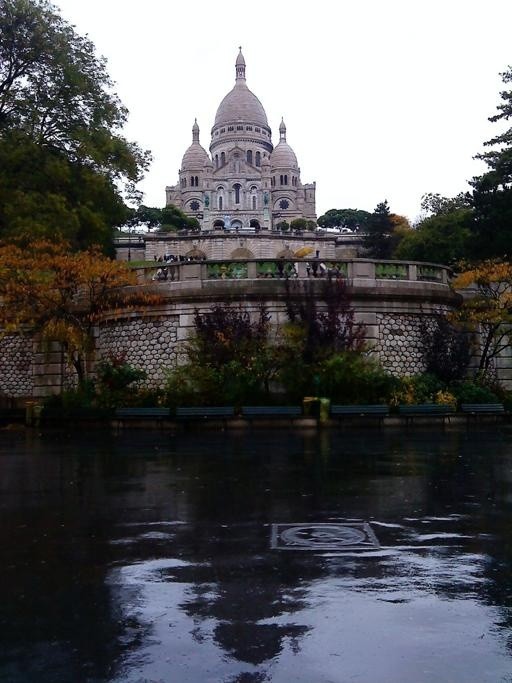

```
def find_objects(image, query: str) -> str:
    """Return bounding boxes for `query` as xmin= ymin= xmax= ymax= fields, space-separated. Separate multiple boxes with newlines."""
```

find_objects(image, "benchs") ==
xmin=114 ymin=407 xmax=172 ymax=440
xmin=175 ymin=406 xmax=240 ymax=432
xmin=42 ymin=405 xmax=114 ymax=445
xmin=329 ymin=404 xmax=391 ymax=433
xmin=397 ymin=404 xmax=456 ymax=437
xmin=240 ymin=405 xmax=303 ymax=433
xmin=461 ymin=403 xmax=506 ymax=432
